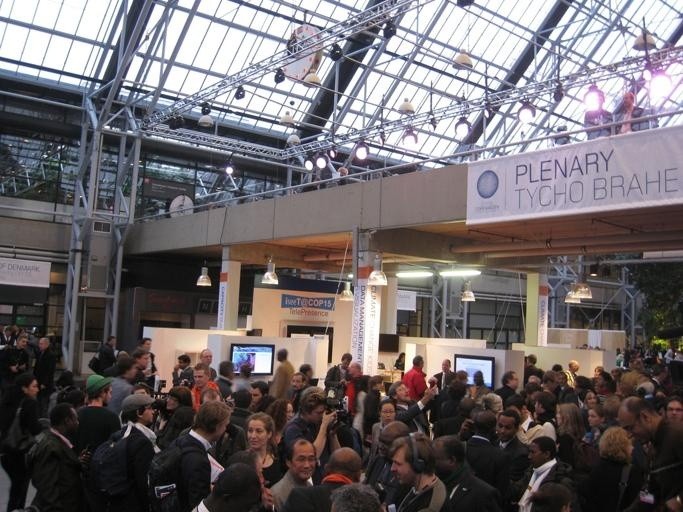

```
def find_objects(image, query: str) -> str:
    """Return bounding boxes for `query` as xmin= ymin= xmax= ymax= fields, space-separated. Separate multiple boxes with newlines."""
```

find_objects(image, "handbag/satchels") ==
xmin=88 ymin=357 xmax=100 ymax=373
xmin=3 ymin=417 xmax=34 ymax=455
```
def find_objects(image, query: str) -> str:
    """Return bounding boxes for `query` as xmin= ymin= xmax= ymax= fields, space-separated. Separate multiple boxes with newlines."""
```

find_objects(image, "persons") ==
xmin=614 ymin=93 xmax=648 ymax=135
xmin=337 ymin=167 xmax=351 ymax=184
xmin=2 ymin=328 xmax=683 ymax=512
xmin=302 ymin=175 xmax=323 ymax=191
xmin=584 ymin=90 xmax=612 ymax=141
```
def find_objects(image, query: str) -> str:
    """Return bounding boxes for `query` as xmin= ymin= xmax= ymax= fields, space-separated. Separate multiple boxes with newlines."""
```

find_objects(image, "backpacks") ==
xmin=147 ymin=435 xmax=206 ymax=512
xmin=86 ymin=429 xmax=144 ymax=497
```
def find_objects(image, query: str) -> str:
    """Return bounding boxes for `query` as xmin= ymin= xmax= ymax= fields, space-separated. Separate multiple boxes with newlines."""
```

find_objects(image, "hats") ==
xmin=56 ymin=371 xmax=74 ymax=385
xmin=121 ymin=394 xmax=156 ymax=412
xmin=86 ymin=375 xmax=112 ymax=395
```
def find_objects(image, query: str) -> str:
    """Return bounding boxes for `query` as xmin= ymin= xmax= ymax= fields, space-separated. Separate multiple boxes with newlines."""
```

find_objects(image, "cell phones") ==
xmin=430 ymin=382 xmax=435 ymax=389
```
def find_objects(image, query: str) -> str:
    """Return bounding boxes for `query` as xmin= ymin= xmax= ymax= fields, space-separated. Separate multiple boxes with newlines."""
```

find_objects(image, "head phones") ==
xmin=409 ymin=432 xmax=426 ymax=474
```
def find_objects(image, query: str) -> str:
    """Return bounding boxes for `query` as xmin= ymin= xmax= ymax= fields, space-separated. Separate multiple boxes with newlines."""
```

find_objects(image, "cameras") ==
xmin=152 ymin=400 xmax=171 ymax=420
xmin=325 ymin=398 xmax=340 ymax=414
xmin=184 ymin=381 xmax=193 ymax=388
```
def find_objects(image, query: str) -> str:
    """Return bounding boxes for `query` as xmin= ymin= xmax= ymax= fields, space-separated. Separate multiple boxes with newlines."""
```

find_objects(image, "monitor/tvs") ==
xmin=231 ymin=344 xmax=275 ymax=376
xmin=454 ymin=354 xmax=495 ymax=391
xmin=379 ymin=334 xmax=399 ymax=352
